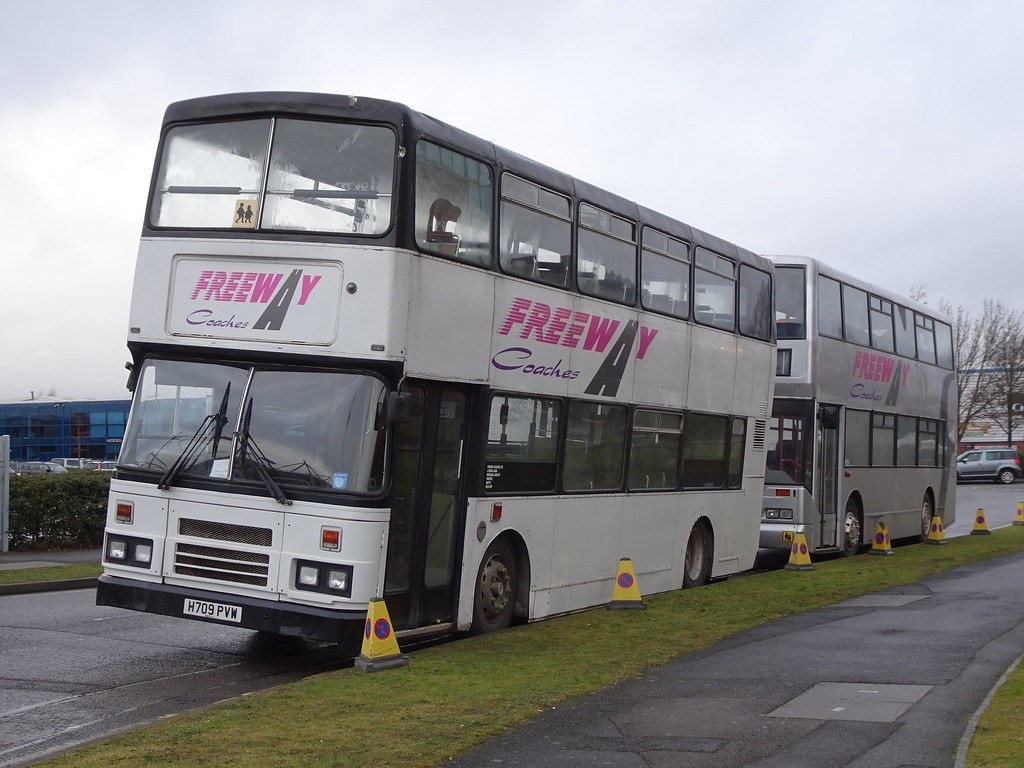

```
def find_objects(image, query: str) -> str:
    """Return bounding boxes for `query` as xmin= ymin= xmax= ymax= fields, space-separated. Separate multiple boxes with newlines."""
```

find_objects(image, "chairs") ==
xmin=578 ymin=469 xmax=667 ymax=489
xmin=420 ymin=231 xmax=751 ymax=336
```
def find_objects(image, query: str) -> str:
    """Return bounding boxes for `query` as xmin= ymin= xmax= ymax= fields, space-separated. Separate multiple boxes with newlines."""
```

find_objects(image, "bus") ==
xmin=665 ymin=256 xmax=961 ymax=552
xmin=95 ymin=89 xmax=778 ymax=648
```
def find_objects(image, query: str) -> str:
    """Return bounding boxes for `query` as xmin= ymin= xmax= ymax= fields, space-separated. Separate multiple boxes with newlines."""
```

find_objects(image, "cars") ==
xmin=10 ymin=461 xmax=69 ymax=476
xmin=956 ymin=448 xmax=1023 ymax=485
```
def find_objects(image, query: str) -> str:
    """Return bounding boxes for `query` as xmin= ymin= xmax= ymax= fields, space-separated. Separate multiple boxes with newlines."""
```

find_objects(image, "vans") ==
xmin=52 ymin=458 xmax=91 ymax=470
xmin=84 ymin=461 xmax=117 ymax=471
